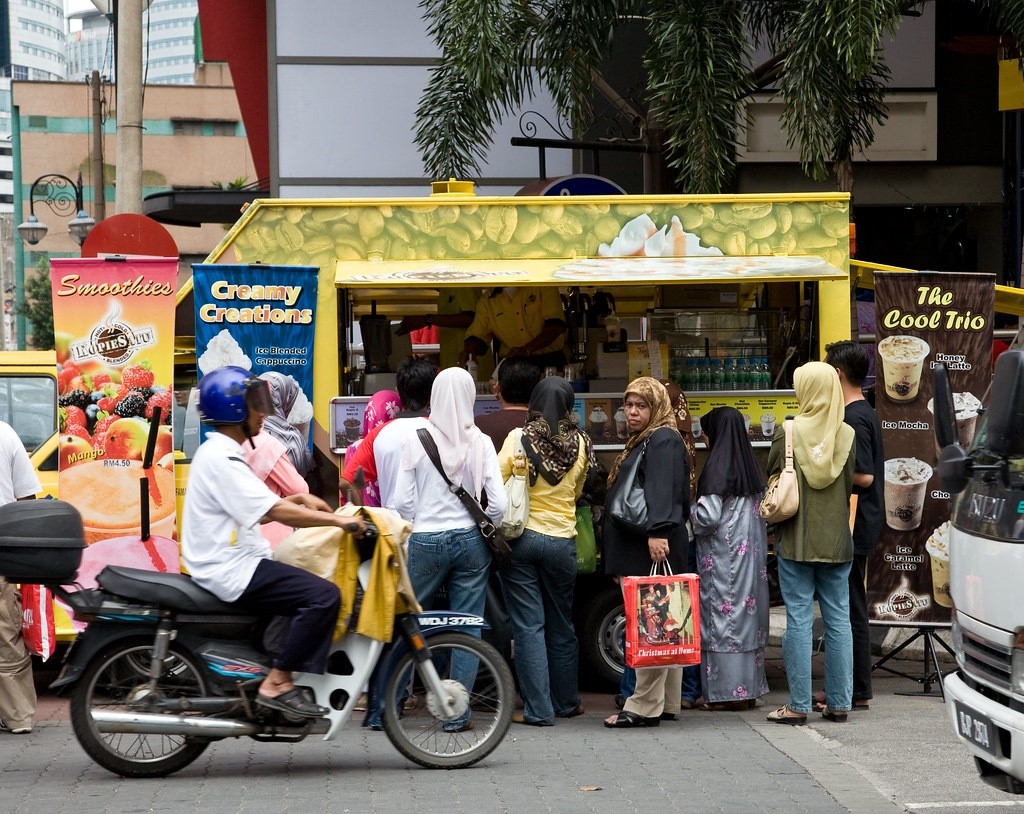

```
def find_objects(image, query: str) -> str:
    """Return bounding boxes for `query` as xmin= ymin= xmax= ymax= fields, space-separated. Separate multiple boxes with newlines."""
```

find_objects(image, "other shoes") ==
xmin=614 ymin=694 xmax=625 ymax=709
xmin=404 ymin=695 xmax=417 ymax=710
xmin=699 ymin=701 xmax=738 ymax=711
xmin=463 ymin=721 xmax=474 ymax=730
xmin=571 ymin=705 xmax=585 ymax=716
xmin=512 ymin=710 xmax=526 ymax=723
xmin=11 ymin=724 xmax=33 ymax=733
xmin=0 ymin=720 xmax=9 ymax=730
xmin=352 ymin=693 xmax=368 ymax=710
xmin=681 ymin=700 xmax=692 ymax=709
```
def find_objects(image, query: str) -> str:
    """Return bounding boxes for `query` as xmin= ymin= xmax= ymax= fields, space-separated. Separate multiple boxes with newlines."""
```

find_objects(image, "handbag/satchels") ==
xmin=620 ymin=558 xmax=702 ymax=670
xmin=760 ymin=422 xmax=799 ymax=524
xmin=477 ymin=516 xmax=512 ymax=564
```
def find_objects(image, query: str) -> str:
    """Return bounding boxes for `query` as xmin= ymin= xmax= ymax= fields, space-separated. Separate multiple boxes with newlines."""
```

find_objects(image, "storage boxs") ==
xmin=654 ymin=284 xmax=741 ymax=309
xmin=761 ymin=282 xmax=796 ymax=309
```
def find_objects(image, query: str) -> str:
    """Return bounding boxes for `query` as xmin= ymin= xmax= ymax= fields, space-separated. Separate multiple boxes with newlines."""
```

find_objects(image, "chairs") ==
xmin=3 ymin=411 xmax=46 ymax=452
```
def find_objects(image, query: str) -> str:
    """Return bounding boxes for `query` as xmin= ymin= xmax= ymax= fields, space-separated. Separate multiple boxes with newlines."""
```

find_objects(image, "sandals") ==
xmin=812 ymin=699 xmax=870 ymax=710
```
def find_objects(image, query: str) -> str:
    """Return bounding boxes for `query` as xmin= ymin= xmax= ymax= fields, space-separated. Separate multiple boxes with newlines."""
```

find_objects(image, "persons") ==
xmin=394 ymin=286 xmax=495 ymax=381
xmin=687 ymin=406 xmax=771 ymax=713
xmin=811 ymin=340 xmax=884 ymax=712
xmin=462 ymin=284 xmax=568 ymax=377
xmin=240 ymin=371 xmax=314 ymax=555
xmin=0 ymin=419 xmax=43 ymax=732
xmin=180 ymin=366 xmax=368 ymax=718
xmin=767 ymin=361 xmax=854 ymax=723
xmin=341 ymin=352 xmax=698 ymax=732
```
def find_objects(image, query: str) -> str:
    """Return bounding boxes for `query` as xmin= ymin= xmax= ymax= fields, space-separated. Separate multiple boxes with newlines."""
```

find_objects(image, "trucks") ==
xmin=930 ymin=309 xmax=1024 ymax=789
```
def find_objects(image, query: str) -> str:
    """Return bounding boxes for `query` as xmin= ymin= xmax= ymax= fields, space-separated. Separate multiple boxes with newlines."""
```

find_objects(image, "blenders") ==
xmin=359 ymin=299 xmax=406 ymax=395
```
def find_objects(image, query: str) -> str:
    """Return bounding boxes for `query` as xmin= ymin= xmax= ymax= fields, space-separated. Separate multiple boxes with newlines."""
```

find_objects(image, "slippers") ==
xmin=822 ymin=705 xmax=847 ymax=722
xmin=254 ymin=687 xmax=331 ymax=718
xmin=660 ymin=712 xmax=675 ymax=720
xmin=765 ymin=704 xmax=807 ymax=726
xmin=603 ymin=711 xmax=660 ymax=727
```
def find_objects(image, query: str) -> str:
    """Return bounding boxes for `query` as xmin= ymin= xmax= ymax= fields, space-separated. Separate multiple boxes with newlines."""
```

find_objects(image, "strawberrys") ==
xmin=57 ymin=358 xmax=173 ymax=461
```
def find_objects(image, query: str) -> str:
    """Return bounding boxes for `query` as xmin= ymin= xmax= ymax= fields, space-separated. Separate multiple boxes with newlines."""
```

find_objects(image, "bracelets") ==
xmin=428 ymin=315 xmax=433 ymax=328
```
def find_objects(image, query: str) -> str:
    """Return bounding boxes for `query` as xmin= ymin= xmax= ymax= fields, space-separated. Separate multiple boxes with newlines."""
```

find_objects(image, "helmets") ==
xmin=197 ymin=366 xmax=268 ymax=425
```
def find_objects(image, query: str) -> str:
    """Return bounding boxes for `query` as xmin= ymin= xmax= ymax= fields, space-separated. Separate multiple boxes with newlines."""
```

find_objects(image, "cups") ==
xmin=565 ymin=366 xmax=575 ymax=381
xmin=546 ymin=367 xmax=556 ymax=377
xmin=925 ymin=534 xmax=955 ymax=607
xmin=927 ymin=392 xmax=983 ymax=461
xmin=476 ymin=382 xmax=491 ymax=394
xmin=884 ymin=457 xmax=933 ymax=530
xmin=614 ymin=420 xmax=629 ymax=439
xmin=878 ymin=335 xmax=931 ymax=404
xmin=343 ymin=419 xmax=361 ymax=443
xmin=590 ymin=421 xmax=607 ymax=442
xmin=691 ymin=415 xmax=702 ymax=438
xmin=58 ymin=458 xmax=177 ymax=545
xmin=743 ymin=414 xmax=750 ymax=433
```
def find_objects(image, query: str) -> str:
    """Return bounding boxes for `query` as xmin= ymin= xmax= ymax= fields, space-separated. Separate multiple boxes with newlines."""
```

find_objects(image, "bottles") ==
xmin=737 ymin=358 xmax=750 ymax=390
xmin=465 ymin=353 xmax=478 ymax=380
xmin=724 ymin=358 xmax=738 ymax=390
xmin=760 ymin=415 xmax=776 ymax=436
xmin=698 ymin=358 xmax=711 ymax=391
xmin=760 ymin=358 xmax=771 ymax=390
xmin=711 ymin=359 xmax=725 ymax=390
xmin=670 ymin=361 xmax=686 ymax=391
xmin=684 ymin=358 xmax=700 ymax=391
xmin=750 ymin=358 xmax=760 ymax=390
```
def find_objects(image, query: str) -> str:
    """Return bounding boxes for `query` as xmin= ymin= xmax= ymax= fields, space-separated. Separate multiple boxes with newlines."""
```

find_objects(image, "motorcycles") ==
xmin=0 ymin=498 xmax=516 ymax=778
